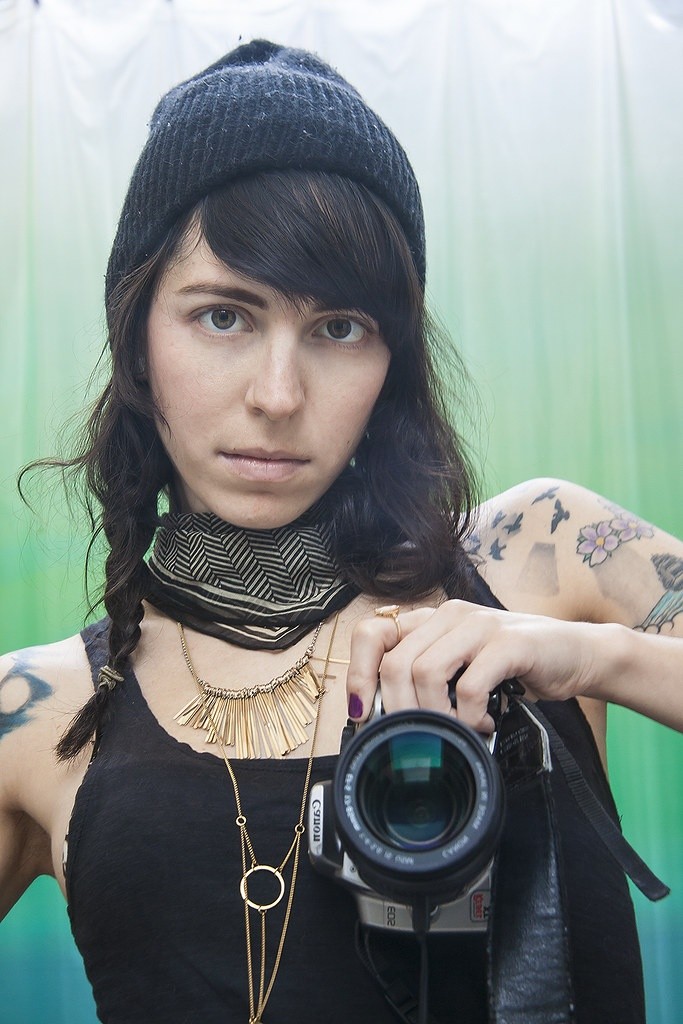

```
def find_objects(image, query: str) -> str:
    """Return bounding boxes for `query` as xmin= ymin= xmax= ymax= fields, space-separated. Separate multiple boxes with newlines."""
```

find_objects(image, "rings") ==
xmin=375 ymin=605 xmax=401 ymax=642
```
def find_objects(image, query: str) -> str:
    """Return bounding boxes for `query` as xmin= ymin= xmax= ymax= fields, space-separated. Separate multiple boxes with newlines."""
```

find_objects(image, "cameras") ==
xmin=309 ymin=662 xmax=506 ymax=934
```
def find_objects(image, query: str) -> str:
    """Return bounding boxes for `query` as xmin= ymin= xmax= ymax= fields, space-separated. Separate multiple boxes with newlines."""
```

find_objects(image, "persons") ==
xmin=0 ymin=38 xmax=683 ymax=1023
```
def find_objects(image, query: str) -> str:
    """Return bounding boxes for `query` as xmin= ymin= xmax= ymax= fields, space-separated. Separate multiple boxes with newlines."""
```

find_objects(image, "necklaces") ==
xmin=178 ymin=611 xmax=341 ymax=1024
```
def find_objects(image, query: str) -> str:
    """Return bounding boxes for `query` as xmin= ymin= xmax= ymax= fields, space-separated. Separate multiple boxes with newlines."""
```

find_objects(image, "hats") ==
xmin=104 ymin=38 xmax=426 ymax=338
